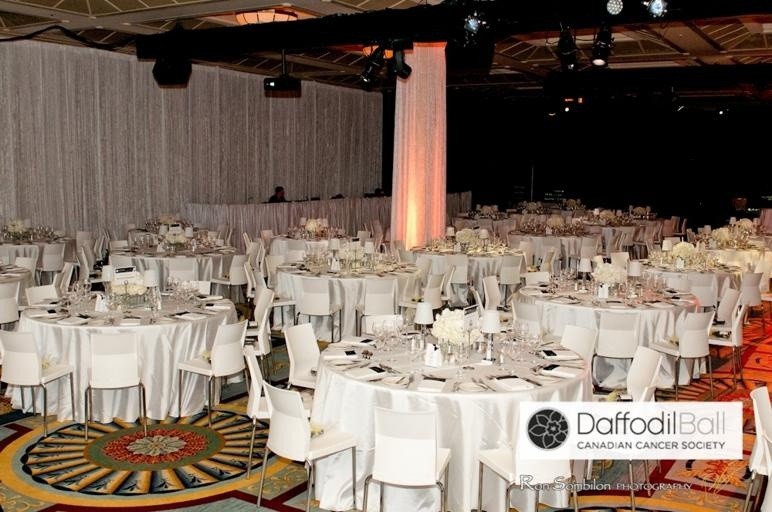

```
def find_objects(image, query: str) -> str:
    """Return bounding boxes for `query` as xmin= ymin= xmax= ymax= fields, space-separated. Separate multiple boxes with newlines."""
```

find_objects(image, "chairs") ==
xmin=1 ymin=198 xmax=772 ymax=512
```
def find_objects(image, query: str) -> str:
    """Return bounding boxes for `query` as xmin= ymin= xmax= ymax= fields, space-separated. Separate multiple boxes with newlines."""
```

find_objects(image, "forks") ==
xmin=471 ymin=377 xmax=495 ymax=391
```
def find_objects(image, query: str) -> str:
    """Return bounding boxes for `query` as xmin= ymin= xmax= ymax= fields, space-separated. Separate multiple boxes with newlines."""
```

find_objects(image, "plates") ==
xmin=279 ymin=266 xmax=296 ymax=270
xmin=328 ymin=342 xmax=353 ymax=350
xmin=646 ymin=299 xmax=673 ymax=309
xmin=551 ymin=298 xmax=580 ymax=304
xmin=382 ymin=377 xmax=409 ymax=386
xmin=328 ymin=359 xmax=355 ymax=369
xmin=458 ymin=382 xmax=488 ymax=392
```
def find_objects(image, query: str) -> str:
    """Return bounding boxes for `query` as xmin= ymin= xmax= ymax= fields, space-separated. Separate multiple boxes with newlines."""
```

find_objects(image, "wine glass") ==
xmin=558 ymin=267 xmax=667 ymax=304
xmin=301 ymin=243 xmax=400 ymax=274
xmin=648 ymin=249 xmax=720 ymax=270
xmin=371 ymin=315 xmax=542 ymax=371
xmin=133 ymin=234 xmax=215 ymax=255
xmin=62 ymin=277 xmax=200 ymax=318
xmin=4 ymin=223 xmax=53 ymax=241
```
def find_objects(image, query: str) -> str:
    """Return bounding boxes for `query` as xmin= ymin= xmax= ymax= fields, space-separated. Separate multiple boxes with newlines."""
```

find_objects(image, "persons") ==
xmin=269 ymin=186 xmax=287 ymax=201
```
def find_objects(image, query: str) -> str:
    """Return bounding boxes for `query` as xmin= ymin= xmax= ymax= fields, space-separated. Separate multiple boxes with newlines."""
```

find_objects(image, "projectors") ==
xmin=264 ymin=78 xmax=301 ymax=98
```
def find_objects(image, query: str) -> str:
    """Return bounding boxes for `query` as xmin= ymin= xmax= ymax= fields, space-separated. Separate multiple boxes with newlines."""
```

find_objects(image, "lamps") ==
xmin=604 ymin=1 xmax=670 ymax=20
xmin=359 ymin=39 xmax=413 ymax=87
xmin=586 ymin=17 xmax=615 ymax=71
xmin=233 ymin=8 xmax=297 ymax=29
xmin=452 ymin=13 xmax=497 ymax=50
xmin=558 ymin=27 xmax=581 ymax=75
